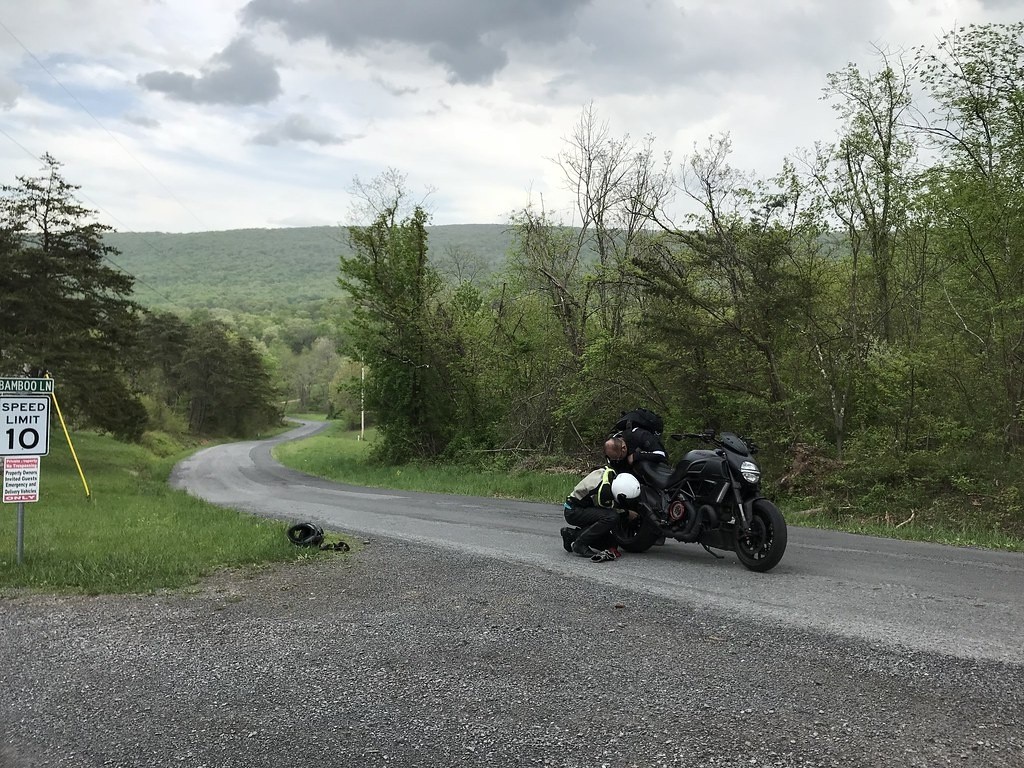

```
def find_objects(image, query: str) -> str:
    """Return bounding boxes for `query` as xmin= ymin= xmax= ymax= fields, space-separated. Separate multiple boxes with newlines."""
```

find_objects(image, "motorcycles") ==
xmin=604 ymin=429 xmax=787 ymax=571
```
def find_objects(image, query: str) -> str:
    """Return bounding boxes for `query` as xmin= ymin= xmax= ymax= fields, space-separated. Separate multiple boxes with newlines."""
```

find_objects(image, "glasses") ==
xmin=610 ymin=444 xmax=622 ymax=461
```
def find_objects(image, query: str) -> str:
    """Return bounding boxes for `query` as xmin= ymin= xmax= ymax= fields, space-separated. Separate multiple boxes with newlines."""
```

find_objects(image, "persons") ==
xmin=559 ymin=464 xmax=641 ymax=557
xmin=604 ymin=427 xmax=669 ymax=464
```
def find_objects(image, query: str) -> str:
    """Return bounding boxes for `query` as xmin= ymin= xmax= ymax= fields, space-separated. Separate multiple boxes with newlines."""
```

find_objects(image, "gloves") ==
xmin=590 ymin=549 xmax=616 ymax=563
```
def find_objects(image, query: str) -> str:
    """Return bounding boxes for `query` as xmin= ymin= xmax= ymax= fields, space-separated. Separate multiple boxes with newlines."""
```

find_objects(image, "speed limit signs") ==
xmin=0 ymin=396 xmax=50 ymax=455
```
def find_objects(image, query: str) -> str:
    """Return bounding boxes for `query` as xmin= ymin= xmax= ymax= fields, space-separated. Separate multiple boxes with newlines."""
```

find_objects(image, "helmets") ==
xmin=611 ymin=473 xmax=641 ymax=503
xmin=287 ymin=522 xmax=324 ymax=548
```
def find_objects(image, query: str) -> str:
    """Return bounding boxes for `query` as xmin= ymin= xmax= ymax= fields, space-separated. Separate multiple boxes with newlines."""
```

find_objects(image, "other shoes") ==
xmin=573 ymin=540 xmax=597 ymax=557
xmin=655 ymin=540 xmax=665 ymax=546
xmin=560 ymin=527 xmax=578 ymax=552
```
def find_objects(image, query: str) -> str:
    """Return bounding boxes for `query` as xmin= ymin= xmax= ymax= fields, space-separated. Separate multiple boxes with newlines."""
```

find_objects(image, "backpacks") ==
xmin=609 ymin=407 xmax=664 ymax=443
